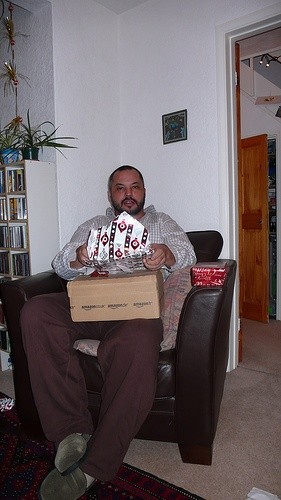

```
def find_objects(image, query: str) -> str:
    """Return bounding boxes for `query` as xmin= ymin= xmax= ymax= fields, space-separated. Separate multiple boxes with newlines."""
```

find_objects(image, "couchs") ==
xmin=0 ymin=229 xmax=238 ymax=469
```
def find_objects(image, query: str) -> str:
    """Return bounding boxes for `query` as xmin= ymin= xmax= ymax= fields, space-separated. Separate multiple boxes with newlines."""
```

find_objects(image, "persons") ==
xmin=20 ymin=165 xmax=198 ymax=500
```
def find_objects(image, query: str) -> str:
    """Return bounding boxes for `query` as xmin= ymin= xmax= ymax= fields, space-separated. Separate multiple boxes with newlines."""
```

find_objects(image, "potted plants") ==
xmin=0 ymin=108 xmax=81 ymax=163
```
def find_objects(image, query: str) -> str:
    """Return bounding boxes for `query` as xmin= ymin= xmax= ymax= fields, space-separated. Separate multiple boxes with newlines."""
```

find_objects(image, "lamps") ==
xmin=265 ymin=57 xmax=274 ymax=67
xmin=259 ymin=53 xmax=265 ymax=64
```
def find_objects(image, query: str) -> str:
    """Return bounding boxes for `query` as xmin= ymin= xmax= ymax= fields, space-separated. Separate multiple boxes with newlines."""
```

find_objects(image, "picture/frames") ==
xmin=162 ymin=109 xmax=187 ymax=145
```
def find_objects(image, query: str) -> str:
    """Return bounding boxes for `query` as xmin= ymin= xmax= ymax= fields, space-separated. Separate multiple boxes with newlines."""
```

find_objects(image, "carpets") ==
xmin=0 ymin=390 xmax=206 ymax=500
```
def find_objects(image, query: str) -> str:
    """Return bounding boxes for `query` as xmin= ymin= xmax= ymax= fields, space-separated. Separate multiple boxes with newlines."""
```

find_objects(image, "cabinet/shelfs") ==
xmin=0 ymin=159 xmax=60 ymax=371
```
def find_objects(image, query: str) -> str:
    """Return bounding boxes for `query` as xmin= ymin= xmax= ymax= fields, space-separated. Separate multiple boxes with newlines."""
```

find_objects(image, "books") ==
xmin=0 ymin=167 xmax=29 ymax=352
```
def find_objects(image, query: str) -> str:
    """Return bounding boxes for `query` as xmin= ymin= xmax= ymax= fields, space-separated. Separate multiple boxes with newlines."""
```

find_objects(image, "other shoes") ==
xmin=38 ymin=467 xmax=97 ymax=500
xmin=55 ymin=432 xmax=92 ymax=476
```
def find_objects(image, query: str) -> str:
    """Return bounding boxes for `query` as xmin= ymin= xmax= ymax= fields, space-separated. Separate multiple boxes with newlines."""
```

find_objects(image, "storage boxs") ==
xmin=65 ymin=268 xmax=168 ymax=324
xmin=191 ymin=261 xmax=228 ymax=287
